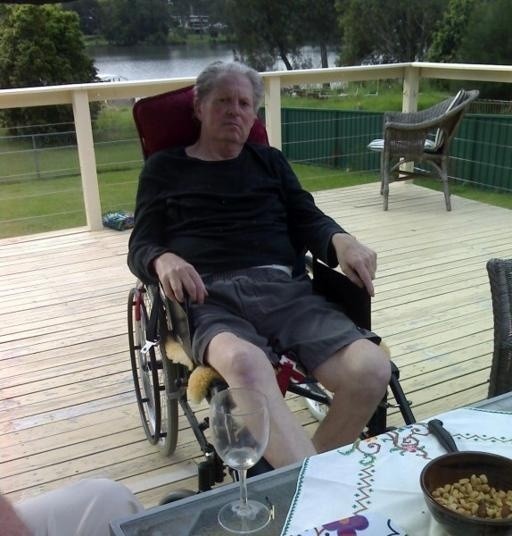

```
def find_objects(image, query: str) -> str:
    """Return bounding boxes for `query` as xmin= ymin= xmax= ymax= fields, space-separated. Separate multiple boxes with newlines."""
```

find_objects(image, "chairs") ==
xmin=486 ymin=255 xmax=511 ymax=401
xmin=369 ymin=85 xmax=480 ymax=212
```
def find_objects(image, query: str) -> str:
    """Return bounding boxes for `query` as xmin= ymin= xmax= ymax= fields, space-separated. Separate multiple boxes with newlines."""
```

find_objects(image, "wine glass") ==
xmin=207 ymin=385 xmax=273 ymax=535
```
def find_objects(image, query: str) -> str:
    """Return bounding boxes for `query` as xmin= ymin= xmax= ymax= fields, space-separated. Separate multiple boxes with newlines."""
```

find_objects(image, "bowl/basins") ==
xmin=419 ymin=451 xmax=512 ymax=536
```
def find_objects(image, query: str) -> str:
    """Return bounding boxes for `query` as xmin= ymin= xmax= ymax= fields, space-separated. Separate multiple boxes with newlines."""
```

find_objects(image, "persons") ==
xmin=126 ymin=59 xmax=392 ymax=470
xmin=0 ymin=478 xmax=145 ymax=536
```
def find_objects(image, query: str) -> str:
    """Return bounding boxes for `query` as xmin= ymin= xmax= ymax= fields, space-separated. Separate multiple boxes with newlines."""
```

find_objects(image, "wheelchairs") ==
xmin=128 ymin=251 xmax=416 ymax=506
